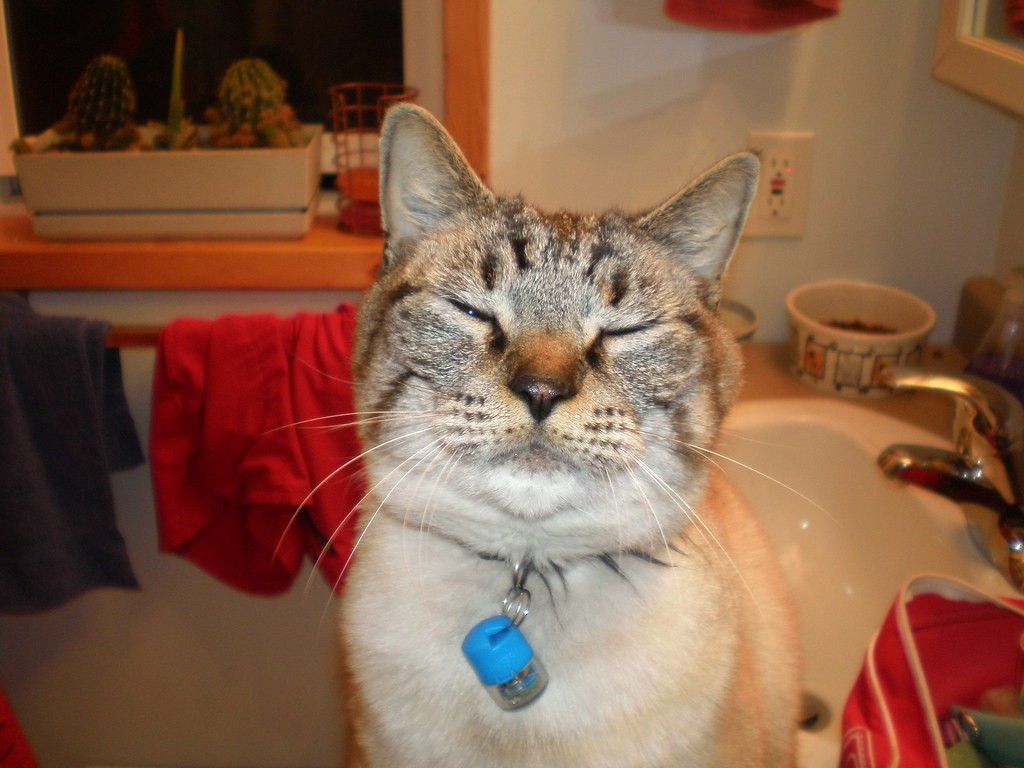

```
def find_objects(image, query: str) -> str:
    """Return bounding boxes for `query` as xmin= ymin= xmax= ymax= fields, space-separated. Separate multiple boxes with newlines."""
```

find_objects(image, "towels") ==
xmin=0 ymin=289 xmax=147 ymax=613
xmin=146 ymin=304 xmax=366 ymax=600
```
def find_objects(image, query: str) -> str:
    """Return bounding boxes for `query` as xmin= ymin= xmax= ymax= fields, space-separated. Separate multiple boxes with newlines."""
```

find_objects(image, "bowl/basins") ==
xmin=718 ymin=299 xmax=758 ymax=362
xmin=787 ymin=279 xmax=936 ymax=401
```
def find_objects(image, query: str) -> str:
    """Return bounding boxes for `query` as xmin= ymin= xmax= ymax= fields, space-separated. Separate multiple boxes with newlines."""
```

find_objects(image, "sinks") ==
xmin=694 ymin=397 xmax=1024 ymax=768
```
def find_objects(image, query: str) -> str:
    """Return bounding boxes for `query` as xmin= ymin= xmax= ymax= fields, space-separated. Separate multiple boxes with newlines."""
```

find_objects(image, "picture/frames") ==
xmin=929 ymin=0 xmax=1024 ymax=118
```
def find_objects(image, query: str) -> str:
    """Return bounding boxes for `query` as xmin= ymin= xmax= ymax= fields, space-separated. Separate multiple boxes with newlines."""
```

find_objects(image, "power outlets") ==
xmin=741 ymin=128 xmax=814 ymax=240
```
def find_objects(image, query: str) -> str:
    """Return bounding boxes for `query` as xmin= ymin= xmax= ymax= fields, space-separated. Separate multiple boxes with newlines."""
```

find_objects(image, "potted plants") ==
xmin=11 ymin=57 xmax=324 ymax=239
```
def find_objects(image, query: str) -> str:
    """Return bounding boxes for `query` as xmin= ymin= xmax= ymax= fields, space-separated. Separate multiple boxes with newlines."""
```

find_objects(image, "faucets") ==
xmin=877 ymin=364 xmax=1024 ymax=595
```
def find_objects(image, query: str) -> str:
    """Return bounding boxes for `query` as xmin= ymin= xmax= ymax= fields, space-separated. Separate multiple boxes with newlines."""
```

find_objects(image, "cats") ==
xmin=255 ymin=102 xmax=840 ymax=768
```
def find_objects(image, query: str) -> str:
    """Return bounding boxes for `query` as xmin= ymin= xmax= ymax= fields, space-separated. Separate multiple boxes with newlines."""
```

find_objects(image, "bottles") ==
xmin=965 ymin=267 xmax=1024 ymax=407
